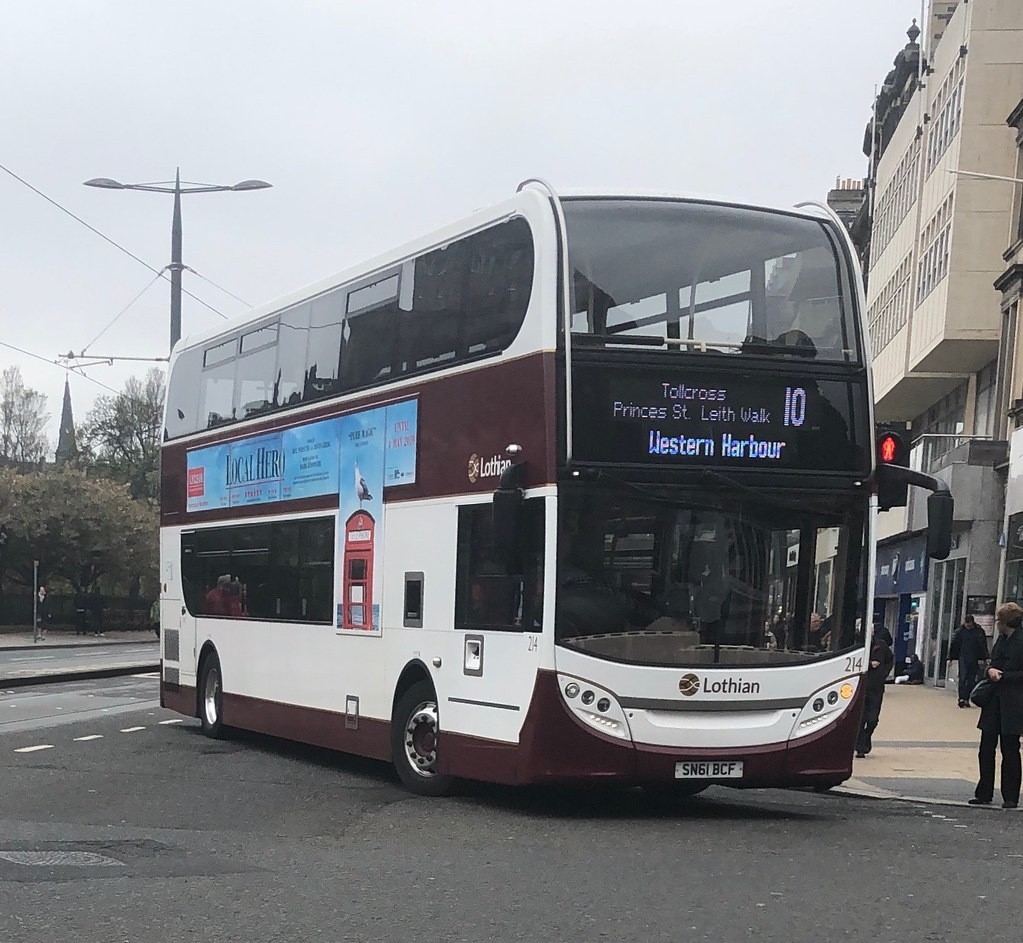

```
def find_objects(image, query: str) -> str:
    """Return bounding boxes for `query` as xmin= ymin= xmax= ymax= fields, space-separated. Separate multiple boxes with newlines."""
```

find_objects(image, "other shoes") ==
xmin=1001 ymin=801 xmax=1017 ymax=808
xmin=959 ymin=699 xmax=969 ymax=707
xmin=969 ymin=798 xmax=990 ymax=804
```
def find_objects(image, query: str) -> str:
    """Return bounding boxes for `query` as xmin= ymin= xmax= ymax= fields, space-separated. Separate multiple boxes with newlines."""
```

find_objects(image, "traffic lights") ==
xmin=876 ymin=430 xmax=908 ymax=507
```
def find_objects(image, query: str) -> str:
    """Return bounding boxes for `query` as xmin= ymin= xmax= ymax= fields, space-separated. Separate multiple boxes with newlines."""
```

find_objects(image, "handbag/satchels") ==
xmin=970 ymin=679 xmax=995 ymax=706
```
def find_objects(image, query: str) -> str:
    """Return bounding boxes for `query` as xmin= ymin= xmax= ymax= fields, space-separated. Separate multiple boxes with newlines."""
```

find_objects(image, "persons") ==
xmin=948 ymin=615 xmax=987 ymax=709
xmin=765 ymin=612 xmax=785 ymax=649
xmin=73 ymin=586 xmax=106 ymax=637
xmin=968 ymin=602 xmax=1023 ymax=808
xmin=809 ymin=612 xmax=832 ymax=650
xmin=903 ymin=654 xmax=923 ymax=684
xmin=149 ymin=595 xmax=160 ymax=637
xmin=36 ymin=587 xmax=51 ymax=641
xmin=522 ymin=538 xmax=593 ymax=601
xmin=204 ymin=573 xmax=242 ymax=616
xmin=854 ymin=627 xmax=894 ymax=757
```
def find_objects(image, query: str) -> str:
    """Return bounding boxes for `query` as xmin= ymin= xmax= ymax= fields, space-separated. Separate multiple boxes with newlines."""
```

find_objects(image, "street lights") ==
xmin=84 ymin=177 xmax=274 ymax=358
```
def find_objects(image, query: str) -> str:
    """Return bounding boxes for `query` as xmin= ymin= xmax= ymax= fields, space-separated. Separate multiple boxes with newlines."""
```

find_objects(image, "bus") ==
xmin=160 ymin=183 xmax=954 ymax=800
